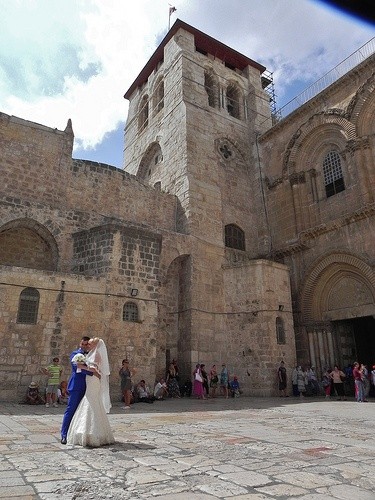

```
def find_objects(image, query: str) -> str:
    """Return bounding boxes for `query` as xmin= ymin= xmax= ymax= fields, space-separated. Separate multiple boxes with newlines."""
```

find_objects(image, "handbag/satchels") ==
xmin=195 ymin=368 xmax=203 ymax=382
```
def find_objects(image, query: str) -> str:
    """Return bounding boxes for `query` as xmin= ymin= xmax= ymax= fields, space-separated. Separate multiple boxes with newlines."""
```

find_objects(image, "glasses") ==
xmin=55 ymin=360 xmax=59 ymax=362
xmin=125 ymin=362 xmax=129 ymax=364
xmin=222 ymin=365 xmax=225 ymax=366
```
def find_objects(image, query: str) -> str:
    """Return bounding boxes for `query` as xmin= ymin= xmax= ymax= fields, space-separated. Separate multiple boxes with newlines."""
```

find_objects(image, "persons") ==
xmin=278 ymin=361 xmax=375 ymax=403
xmin=61 ymin=336 xmax=97 ymax=444
xmin=27 ymin=358 xmax=244 ymax=409
xmin=66 ymin=337 xmax=116 ymax=447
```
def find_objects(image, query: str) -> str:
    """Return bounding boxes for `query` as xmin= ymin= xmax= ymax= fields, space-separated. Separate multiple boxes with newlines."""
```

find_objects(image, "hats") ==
xmin=28 ymin=382 xmax=37 ymax=388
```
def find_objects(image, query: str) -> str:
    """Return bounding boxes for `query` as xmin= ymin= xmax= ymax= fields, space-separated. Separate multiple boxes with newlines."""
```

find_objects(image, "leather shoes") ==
xmin=60 ymin=437 xmax=68 ymax=444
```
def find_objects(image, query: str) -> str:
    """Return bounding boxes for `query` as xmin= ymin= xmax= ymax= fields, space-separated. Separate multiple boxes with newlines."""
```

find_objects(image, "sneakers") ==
xmin=120 ymin=406 xmax=131 ymax=410
